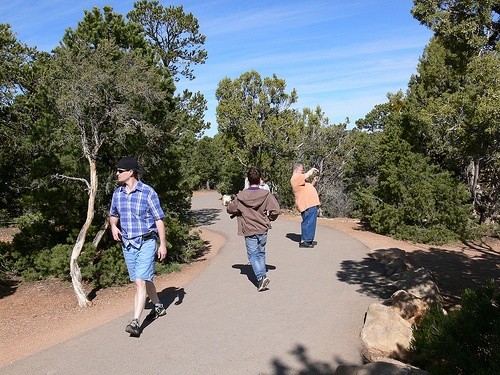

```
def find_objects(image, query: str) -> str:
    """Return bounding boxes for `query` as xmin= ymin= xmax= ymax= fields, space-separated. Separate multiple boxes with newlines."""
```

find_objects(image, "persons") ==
xmin=290 ymin=162 xmax=321 ymax=248
xmin=227 ymin=167 xmax=280 ymax=292
xmin=258 ymin=176 xmax=270 ymax=192
xmin=109 ymin=156 xmax=167 ymax=337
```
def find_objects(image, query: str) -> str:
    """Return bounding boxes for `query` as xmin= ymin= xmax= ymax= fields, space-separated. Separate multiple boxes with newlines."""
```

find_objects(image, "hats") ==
xmin=116 ymin=158 xmax=144 ymax=173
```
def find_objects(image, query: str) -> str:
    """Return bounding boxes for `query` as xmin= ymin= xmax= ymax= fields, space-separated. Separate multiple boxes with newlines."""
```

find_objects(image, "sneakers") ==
xmin=299 ymin=241 xmax=317 ymax=248
xmin=146 ymin=304 xmax=166 ymax=320
xmin=258 ymin=275 xmax=270 ymax=292
xmin=126 ymin=318 xmax=140 ymax=336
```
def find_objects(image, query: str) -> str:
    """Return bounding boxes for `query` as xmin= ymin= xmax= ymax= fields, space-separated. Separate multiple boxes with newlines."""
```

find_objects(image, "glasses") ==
xmin=117 ymin=170 xmax=126 ymax=173
